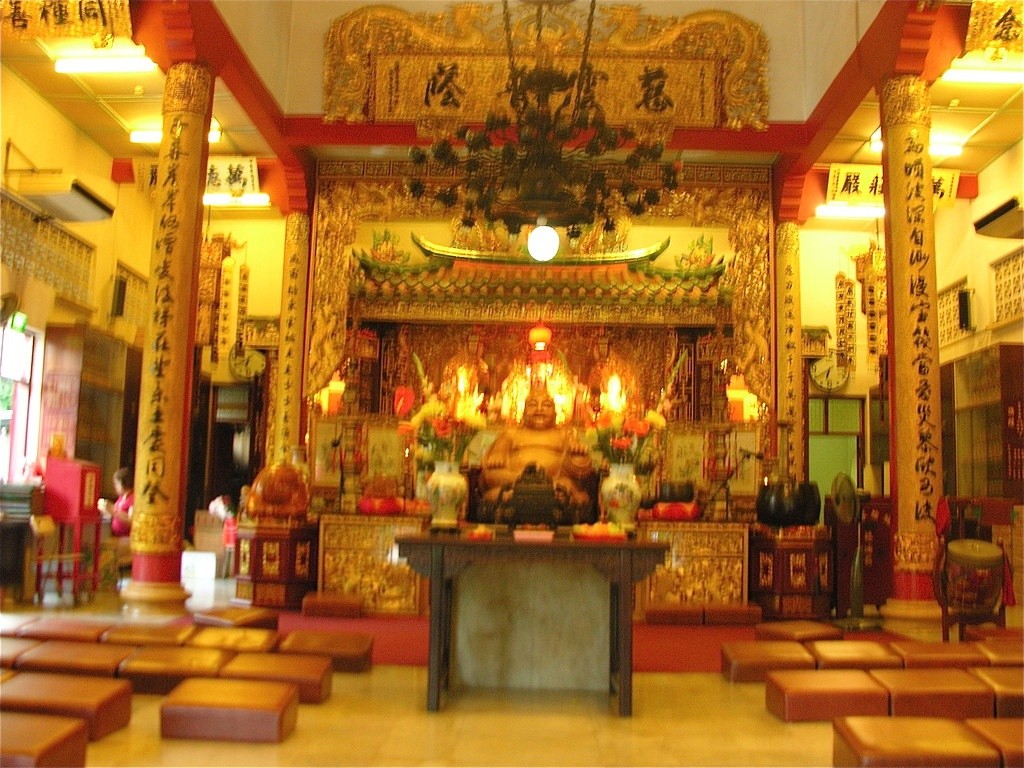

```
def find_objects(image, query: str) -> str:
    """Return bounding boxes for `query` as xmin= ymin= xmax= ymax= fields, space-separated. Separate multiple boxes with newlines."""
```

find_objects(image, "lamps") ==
xmin=406 ymin=0 xmax=685 ymax=239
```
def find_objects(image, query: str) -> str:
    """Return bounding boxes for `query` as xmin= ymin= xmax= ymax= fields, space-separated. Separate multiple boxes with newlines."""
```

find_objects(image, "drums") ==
xmin=946 ymin=537 xmax=1003 ymax=623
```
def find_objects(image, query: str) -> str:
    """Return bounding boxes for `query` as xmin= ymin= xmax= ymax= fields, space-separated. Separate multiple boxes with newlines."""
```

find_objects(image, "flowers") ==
xmin=591 ymin=395 xmax=665 ymax=469
xmin=395 ymin=352 xmax=491 ymax=461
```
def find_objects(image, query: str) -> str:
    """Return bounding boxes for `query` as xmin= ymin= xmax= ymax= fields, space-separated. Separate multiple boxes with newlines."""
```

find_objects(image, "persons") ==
xmin=97 ymin=467 xmax=134 ymax=579
xmin=478 ymin=388 xmax=591 ymax=517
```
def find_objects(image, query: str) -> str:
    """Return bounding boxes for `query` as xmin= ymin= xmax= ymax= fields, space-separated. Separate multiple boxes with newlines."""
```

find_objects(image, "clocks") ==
xmin=228 ymin=343 xmax=266 ymax=383
xmin=809 ymin=348 xmax=851 ymax=393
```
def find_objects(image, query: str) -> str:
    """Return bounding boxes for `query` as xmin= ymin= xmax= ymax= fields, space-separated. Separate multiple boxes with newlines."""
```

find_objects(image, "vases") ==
xmin=600 ymin=461 xmax=641 ymax=533
xmin=424 ymin=461 xmax=468 ymax=529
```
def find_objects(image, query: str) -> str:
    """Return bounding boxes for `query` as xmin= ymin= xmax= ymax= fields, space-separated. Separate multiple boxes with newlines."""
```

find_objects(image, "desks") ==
xmin=393 ymin=535 xmax=671 ymax=718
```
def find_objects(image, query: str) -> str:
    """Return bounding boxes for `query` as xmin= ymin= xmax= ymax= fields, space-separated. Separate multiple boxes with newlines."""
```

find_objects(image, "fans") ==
xmin=830 ymin=470 xmax=884 ymax=633
